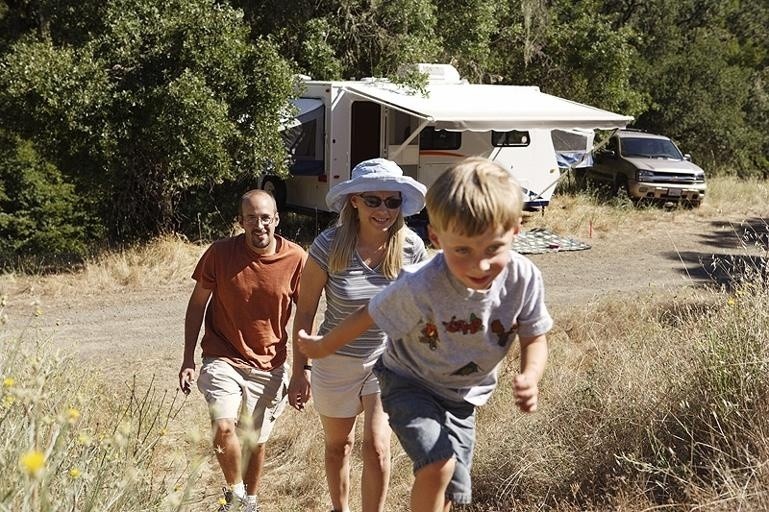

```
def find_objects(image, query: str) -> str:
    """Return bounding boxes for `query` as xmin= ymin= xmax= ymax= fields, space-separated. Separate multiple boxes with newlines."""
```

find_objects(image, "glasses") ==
xmin=360 ymin=191 xmax=403 ymax=209
xmin=241 ymin=215 xmax=275 ymax=225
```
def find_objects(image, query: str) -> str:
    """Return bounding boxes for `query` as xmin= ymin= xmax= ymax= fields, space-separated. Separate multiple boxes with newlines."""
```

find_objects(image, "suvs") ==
xmin=572 ymin=129 xmax=707 ymax=209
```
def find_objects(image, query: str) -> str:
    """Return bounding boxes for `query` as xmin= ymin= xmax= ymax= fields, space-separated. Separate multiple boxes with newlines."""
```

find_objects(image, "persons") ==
xmin=287 ymin=158 xmax=431 ymax=512
xmin=178 ymin=189 xmax=311 ymax=510
xmin=296 ymin=155 xmax=554 ymax=511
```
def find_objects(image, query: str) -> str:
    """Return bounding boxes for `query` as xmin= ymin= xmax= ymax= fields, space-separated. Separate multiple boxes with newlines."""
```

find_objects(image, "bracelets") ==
xmin=303 ymin=364 xmax=312 ymax=371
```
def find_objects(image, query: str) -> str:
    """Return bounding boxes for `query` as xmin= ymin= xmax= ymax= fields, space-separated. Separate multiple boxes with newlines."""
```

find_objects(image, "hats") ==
xmin=326 ymin=159 xmax=427 ymax=218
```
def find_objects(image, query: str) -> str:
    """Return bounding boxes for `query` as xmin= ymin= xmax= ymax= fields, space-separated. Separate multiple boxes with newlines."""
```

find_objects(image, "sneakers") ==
xmin=247 ymin=501 xmax=258 ymax=511
xmin=219 ymin=489 xmax=247 ymax=511
